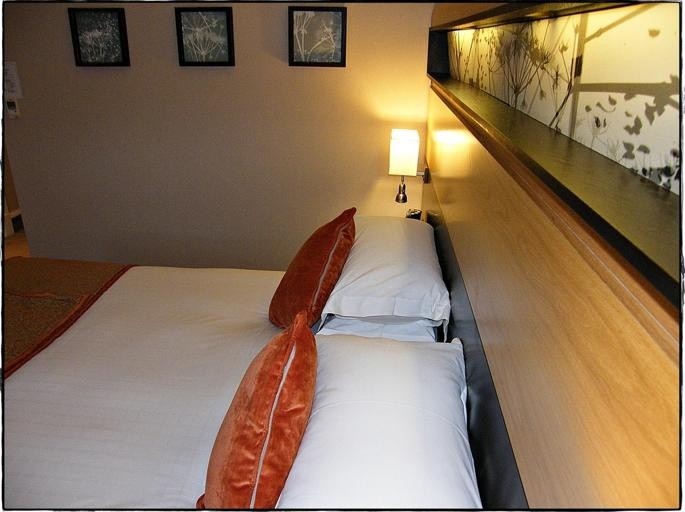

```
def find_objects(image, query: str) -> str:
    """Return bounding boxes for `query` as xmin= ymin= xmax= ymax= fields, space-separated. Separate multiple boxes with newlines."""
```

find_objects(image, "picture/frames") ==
xmin=67 ymin=7 xmax=347 ymax=68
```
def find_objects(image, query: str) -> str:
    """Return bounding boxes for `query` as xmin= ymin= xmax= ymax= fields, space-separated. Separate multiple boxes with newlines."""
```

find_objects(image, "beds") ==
xmin=2 ymin=207 xmax=527 ymax=510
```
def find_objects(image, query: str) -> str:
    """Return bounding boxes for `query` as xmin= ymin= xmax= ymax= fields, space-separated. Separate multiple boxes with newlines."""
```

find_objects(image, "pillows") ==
xmin=271 ymin=335 xmax=483 ymax=509
xmin=195 ymin=309 xmax=318 ymax=510
xmin=268 ymin=206 xmax=359 ymax=328
xmin=325 ymin=214 xmax=450 ymax=323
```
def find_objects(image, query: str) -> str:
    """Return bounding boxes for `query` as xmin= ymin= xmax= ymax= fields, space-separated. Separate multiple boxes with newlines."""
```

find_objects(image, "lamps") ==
xmin=389 ymin=129 xmax=429 ymax=203
